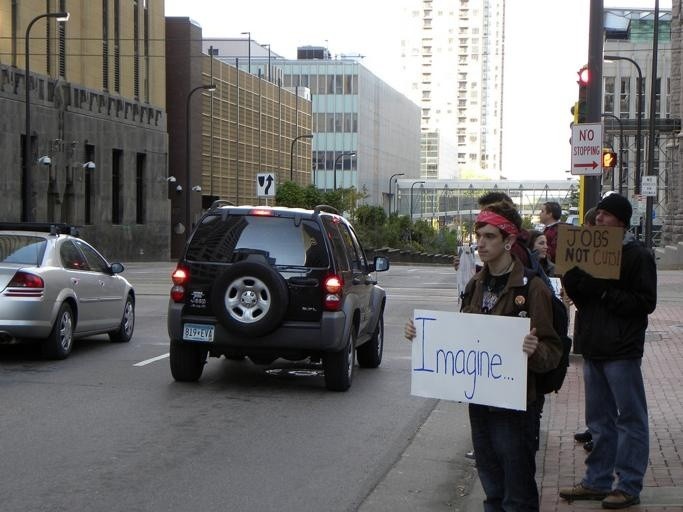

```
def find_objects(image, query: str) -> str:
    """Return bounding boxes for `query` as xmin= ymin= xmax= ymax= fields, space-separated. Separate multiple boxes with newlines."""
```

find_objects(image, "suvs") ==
xmin=165 ymin=197 xmax=390 ymax=393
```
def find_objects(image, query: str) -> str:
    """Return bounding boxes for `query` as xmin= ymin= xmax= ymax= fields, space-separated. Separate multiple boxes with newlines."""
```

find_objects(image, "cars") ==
xmin=1 ymin=222 xmax=136 ymax=356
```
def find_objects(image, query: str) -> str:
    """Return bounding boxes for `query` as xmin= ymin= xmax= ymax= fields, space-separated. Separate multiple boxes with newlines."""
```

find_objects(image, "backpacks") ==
xmin=515 ymin=269 xmax=572 ymax=393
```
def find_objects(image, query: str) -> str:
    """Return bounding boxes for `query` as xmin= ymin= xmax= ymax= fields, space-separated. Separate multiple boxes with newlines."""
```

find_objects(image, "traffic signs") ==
xmin=570 ymin=122 xmax=603 ymax=176
xmin=642 ymin=175 xmax=656 ymax=196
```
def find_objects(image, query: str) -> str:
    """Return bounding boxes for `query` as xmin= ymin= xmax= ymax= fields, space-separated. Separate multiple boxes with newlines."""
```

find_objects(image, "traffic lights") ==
xmin=578 ymin=66 xmax=591 ymax=86
xmin=602 ymin=152 xmax=618 ymax=168
xmin=569 ymin=101 xmax=585 ymax=147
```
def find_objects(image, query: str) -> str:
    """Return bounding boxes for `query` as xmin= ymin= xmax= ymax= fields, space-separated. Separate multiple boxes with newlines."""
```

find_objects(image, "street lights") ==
xmin=333 ymin=151 xmax=356 ymax=191
xmin=185 ymin=82 xmax=216 ymax=249
xmin=604 ymin=54 xmax=643 ymax=240
xmin=240 ymin=32 xmax=251 ymax=73
xmin=22 ymin=11 xmax=74 ymax=222
xmin=389 ymin=173 xmax=405 ymax=199
xmin=411 ymin=181 xmax=425 ymax=223
xmin=260 ymin=44 xmax=271 ymax=81
xmin=291 ymin=134 xmax=314 ymax=182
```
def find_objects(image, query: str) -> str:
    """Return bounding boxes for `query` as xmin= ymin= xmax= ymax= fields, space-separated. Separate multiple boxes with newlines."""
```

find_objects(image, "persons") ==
xmin=402 ymin=198 xmax=573 ymax=510
xmin=523 ymin=227 xmax=563 ymax=278
xmin=573 ymin=205 xmax=600 ymax=452
xmin=556 ymin=194 xmax=659 ymax=508
xmin=452 ymin=191 xmax=536 ymax=460
xmin=537 ymin=199 xmax=562 ymax=264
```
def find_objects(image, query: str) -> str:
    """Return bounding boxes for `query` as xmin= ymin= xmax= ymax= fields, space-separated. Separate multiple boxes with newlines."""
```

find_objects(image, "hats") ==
xmin=586 ymin=207 xmax=596 ymax=225
xmin=597 ymin=193 xmax=632 ymax=225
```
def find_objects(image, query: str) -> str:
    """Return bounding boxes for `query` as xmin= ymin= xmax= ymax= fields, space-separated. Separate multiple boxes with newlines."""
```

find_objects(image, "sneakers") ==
xmin=559 ymin=482 xmax=640 ymax=509
xmin=575 ymin=431 xmax=593 ymax=451
